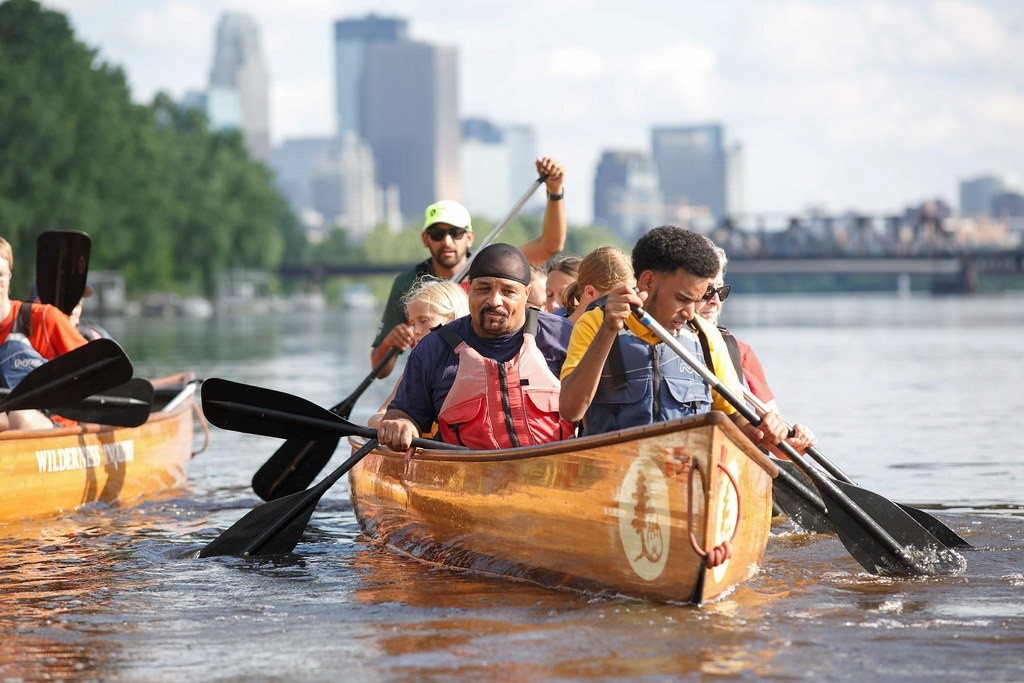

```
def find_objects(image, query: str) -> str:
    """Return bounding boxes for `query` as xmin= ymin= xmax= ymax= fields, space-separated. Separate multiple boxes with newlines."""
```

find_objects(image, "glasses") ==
xmin=428 ymin=227 xmax=465 ymax=241
xmin=527 ymin=300 xmax=547 ymax=312
xmin=702 ymin=286 xmax=730 ymax=301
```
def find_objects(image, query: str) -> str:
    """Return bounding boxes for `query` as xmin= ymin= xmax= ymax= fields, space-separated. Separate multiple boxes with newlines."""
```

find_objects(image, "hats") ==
xmin=33 ymin=276 xmax=93 ymax=299
xmin=422 ymin=200 xmax=471 ymax=232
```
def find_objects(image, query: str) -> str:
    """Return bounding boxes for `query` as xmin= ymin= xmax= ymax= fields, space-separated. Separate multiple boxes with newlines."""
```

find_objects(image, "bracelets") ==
xmin=546 ymin=187 xmax=565 ymax=200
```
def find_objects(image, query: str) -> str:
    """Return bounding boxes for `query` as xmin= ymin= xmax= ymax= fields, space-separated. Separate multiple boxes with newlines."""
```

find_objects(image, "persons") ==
xmin=560 ymin=225 xmax=788 ymax=449
xmin=371 ymin=156 xmax=567 ymax=379
xmin=526 ymin=246 xmax=638 ymax=322
xmin=0 ymin=239 xmax=185 ymax=433
xmin=695 ymin=234 xmax=813 ymax=460
xmin=378 ymin=243 xmax=583 ymax=463
xmin=368 ymin=275 xmax=471 ymax=430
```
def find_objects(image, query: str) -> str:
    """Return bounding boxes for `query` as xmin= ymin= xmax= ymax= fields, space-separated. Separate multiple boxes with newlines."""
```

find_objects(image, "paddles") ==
xmin=2 ymin=376 xmax=157 ymax=428
xmin=757 ymin=450 xmax=841 ymax=538
xmin=737 ymin=380 xmax=975 ymax=554
xmin=1 ymin=337 xmax=136 ymax=414
xmin=33 ymin=228 xmax=94 ymax=319
xmin=194 ymin=439 xmax=378 ymax=561
xmin=250 ymin=164 xmax=545 ymax=503
xmin=198 ymin=374 xmax=479 ymax=453
xmin=629 ymin=285 xmax=952 ymax=576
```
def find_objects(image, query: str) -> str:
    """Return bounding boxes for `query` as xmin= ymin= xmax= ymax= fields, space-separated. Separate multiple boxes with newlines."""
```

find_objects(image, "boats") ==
xmin=1 ymin=372 xmax=198 ymax=525
xmin=346 ymin=411 xmax=774 ymax=604
